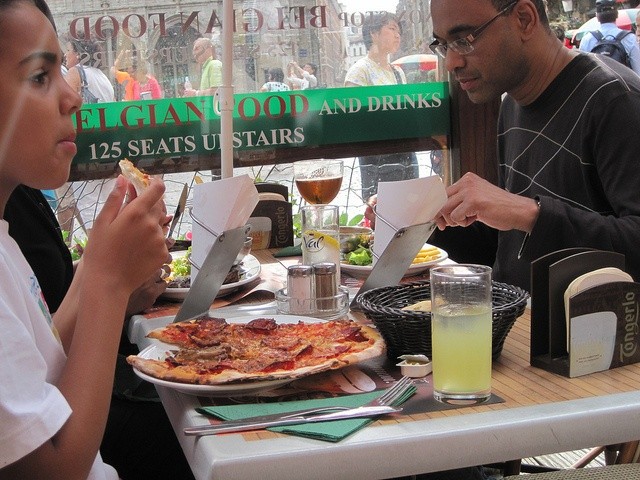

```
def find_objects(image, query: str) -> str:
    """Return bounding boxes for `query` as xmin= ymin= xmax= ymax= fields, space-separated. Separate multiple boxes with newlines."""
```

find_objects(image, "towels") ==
xmin=273 ymin=240 xmax=306 ymax=259
xmin=196 ymin=384 xmax=420 ymax=441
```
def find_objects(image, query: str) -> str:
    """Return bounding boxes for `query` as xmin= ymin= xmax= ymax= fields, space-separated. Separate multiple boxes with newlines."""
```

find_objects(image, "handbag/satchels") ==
xmin=73 ymin=65 xmax=99 ymax=104
xmin=304 ymin=77 xmax=328 ymax=92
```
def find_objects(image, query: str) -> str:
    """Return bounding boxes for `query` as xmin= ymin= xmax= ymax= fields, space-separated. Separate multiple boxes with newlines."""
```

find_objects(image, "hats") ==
xmin=595 ymin=0 xmax=617 ymax=11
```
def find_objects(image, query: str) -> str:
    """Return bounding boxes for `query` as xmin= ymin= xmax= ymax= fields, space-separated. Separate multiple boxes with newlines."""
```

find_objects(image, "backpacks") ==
xmin=589 ymin=29 xmax=633 ymax=72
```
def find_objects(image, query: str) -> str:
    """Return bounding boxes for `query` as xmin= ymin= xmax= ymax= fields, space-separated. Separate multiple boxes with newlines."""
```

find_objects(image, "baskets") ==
xmin=356 ymin=278 xmax=528 ymax=363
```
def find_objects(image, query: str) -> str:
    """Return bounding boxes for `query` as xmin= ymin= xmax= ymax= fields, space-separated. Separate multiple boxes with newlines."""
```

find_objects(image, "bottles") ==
xmin=313 ymin=261 xmax=341 ymax=315
xmin=287 ymin=265 xmax=314 ymax=314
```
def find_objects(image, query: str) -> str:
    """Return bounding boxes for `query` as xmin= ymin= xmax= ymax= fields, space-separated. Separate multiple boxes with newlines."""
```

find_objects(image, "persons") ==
xmin=582 ymin=0 xmax=639 ymax=73
xmin=364 ymin=1 xmax=639 ymax=298
xmin=109 ymin=47 xmax=132 ymax=101
xmin=56 ymin=51 xmax=87 ymax=245
xmin=550 ymin=23 xmax=569 ymax=51
xmin=288 ymin=59 xmax=317 ymax=90
xmin=260 ymin=68 xmax=289 ymax=92
xmin=570 ymin=8 xmax=638 ymax=44
xmin=344 ymin=11 xmax=419 ymax=203
xmin=129 ymin=62 xmax=162 ymax=99
xmin=8 ymin=181 xmax=170 ymax=473
xmin=191 ymin=38 xmax=223 ymax=180
xmin=66 ymin=31 xmax=118 ymax=234
xmin=0 ymin=0 xmax=171 ymax=480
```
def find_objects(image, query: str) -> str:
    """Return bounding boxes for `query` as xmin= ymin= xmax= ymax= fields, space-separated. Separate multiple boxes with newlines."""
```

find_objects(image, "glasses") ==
xmin=128 ymin=69 xmax=138 ymax=76
xmin=429 ymin=1 xmax=519 ymax=59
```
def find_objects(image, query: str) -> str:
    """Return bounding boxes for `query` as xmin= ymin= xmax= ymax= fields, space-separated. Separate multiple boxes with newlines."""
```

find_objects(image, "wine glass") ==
xmin=292 ymin=157 xmax=344 ymax=228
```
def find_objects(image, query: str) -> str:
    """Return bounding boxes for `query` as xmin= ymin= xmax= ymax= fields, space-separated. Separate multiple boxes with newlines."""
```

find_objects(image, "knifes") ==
xmin=183 ymin=405 xmax=405 ymax=437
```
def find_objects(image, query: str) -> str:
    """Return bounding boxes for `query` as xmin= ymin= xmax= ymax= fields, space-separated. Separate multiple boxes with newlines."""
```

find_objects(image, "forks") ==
xmin=221 ymin=374 xmax=416 ymax=427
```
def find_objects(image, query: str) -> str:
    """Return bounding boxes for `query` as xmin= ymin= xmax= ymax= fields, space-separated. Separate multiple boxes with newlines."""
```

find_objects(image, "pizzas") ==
xmin=126 ymin=315 xmax=385 ymax=385
xmin=118 ymin=159 xmax=151 ymax=198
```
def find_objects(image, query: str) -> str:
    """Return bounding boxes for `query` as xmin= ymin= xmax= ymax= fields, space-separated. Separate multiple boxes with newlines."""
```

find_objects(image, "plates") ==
xmin=340 ymin=243 xmax=448 ymax=278
xmin=161 ymin=249 xmax=263 ymax=302
xmin=131 ymin=315 xmax=355 ymax=397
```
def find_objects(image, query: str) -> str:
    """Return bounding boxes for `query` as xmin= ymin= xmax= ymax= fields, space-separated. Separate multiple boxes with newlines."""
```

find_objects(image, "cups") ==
xmin=428 ymin=263 xmax=494 ymax=408
xmin=300 ymin=202 xmax=341 ymax=292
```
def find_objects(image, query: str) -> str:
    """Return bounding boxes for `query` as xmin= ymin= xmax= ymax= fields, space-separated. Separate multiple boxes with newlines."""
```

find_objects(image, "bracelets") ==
xmin=196 ymin=90 xmax=200 ymax=96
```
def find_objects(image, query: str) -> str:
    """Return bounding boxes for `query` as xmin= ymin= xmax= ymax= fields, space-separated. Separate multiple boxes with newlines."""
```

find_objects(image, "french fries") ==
xmin=411 ymin=247 xmax=440 ymax=264
